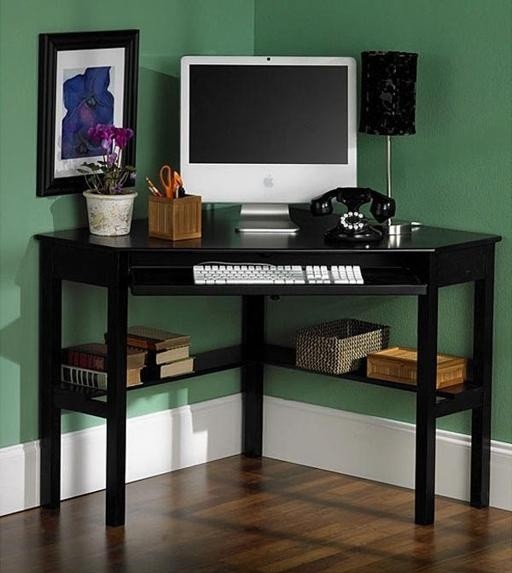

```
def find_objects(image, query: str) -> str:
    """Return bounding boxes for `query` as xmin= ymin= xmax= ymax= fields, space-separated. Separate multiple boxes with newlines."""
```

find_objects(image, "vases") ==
xmin=82 ymin=188 xmax=139 ymax=237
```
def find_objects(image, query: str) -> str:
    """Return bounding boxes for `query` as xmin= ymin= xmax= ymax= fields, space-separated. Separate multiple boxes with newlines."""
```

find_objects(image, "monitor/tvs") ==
xmin=179 ymin=55 xmax=358 ymax=232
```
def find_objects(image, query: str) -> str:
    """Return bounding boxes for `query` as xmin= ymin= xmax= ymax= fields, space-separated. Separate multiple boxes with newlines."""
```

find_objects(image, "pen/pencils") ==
xmin=146 ymin=176 xmax=161 ymax=198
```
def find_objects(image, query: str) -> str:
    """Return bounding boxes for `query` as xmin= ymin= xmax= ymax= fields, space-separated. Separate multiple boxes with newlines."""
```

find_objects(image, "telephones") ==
xmin=311 ymin=187 xmax=395 ymax=243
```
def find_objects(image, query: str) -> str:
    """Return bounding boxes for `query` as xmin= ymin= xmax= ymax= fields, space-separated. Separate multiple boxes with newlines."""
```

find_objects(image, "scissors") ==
xmin=160 ymin=166 xmax=181 ymax=199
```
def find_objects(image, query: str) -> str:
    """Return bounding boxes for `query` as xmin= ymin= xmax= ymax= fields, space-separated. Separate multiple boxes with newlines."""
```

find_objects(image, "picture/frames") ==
xmin=36 ymin=27 xmax=141 ymax=197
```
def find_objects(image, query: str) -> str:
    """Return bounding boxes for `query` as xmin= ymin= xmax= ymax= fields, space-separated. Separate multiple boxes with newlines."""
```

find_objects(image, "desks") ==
xmin=31 ymin=205 xmax=503 ymax=527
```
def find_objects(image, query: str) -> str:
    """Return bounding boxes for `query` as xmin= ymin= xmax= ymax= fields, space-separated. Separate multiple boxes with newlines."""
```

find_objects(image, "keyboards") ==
xmin=193 ymin=264 xmax=364 ymax=285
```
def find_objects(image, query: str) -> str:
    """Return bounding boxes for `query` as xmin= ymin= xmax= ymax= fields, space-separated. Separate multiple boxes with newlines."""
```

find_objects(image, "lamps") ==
xmin=357 ymin=49 xmax=418 ymax=232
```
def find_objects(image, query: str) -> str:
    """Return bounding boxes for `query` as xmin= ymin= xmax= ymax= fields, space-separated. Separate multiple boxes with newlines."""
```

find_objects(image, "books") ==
xmin=61 ymin=325 xmax=193 ymax=391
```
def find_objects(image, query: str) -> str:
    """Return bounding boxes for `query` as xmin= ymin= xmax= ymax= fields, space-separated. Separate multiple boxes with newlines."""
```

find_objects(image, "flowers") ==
xmin=77 ymin=121 xmax=137 ymax=194
